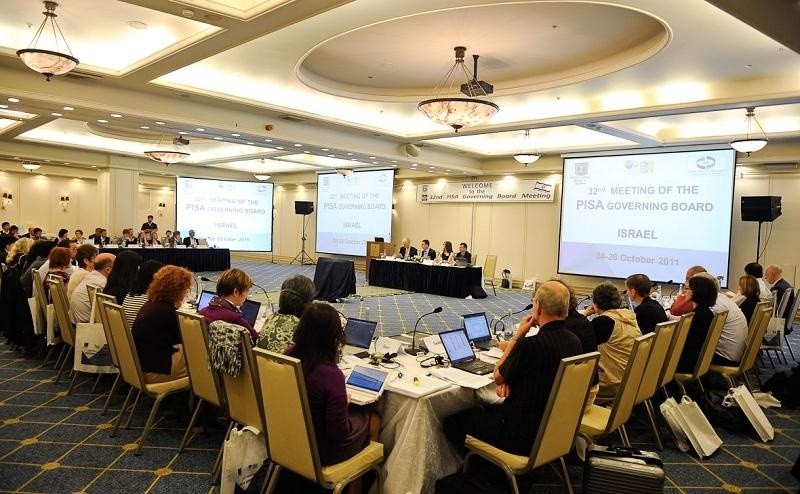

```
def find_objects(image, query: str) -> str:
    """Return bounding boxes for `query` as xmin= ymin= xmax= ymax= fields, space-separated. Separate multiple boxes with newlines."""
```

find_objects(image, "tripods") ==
xmin=289 ymin=214 xmax=316 ymax=265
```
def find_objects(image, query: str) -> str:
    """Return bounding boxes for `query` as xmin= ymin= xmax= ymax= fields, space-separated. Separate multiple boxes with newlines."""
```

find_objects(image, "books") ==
xmin=384 ymin=374 xmax=451 ymax=400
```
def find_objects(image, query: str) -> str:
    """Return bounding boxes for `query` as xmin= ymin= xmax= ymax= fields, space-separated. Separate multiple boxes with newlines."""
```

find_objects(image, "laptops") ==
xmin=239 ymin=299 xmax=260 ymax=329
xmin=341 ymin=317 xmax=377 ymax=355
xmin=438 ymin=328 xmax=497 ymax=376
xmin=454 ymin=257 xmax=467 ymax=266
xmin=344 ymin=365 xmax=391 ymax=406
xmin=461 ymin=312 xmax=499 ymax=351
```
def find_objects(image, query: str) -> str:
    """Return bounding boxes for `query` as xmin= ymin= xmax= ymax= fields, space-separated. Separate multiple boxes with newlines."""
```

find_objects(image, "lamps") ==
xmin=13 ymin=0 xmax=79 ymax=80
xmin=158 ymin=202 xmax=166 ymax=216
xmin=417 ymin=45 xmax=502 ymax=132
xmin=21 ymin=160 xmax=42 ymax=172
xmin=336 ymin=151 xmax=354 ymax=176
xmin=143 ymin=127 xmax=191 ymax=165
xmin=510 ymin=128 xmax=544 ymax=166
xmin=253 ymin=156 xmax=271 ymax=181
xmin=61 ymin=197 xmax=69 ymax=211
xmin=1 ymin=193 xmax=12 ymax=209
xmin=728 ymin=105 xmax=770 ymax=156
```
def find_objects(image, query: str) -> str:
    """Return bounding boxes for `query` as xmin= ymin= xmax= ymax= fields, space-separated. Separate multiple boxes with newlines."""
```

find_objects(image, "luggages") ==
xmin=584 ymin=445 xmax=666 ymax=494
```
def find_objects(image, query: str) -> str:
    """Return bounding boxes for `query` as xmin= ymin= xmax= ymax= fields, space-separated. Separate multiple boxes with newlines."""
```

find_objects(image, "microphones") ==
xmin=404 ymin=306 xmax=442 ymax=356
xmin=187 ymin=274 xmax=274 ymax=313
xmin=576 ymin=279 xmax=684 ymax=311
xmin=492 ymin=304 xmax=533 ymax=336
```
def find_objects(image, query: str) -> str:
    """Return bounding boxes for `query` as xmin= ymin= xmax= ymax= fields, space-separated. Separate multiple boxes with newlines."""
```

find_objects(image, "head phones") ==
xmin=420 ymin=355 xmax=444 ymax=368
xmin=368 ymin=352 xmax=400 ymax=369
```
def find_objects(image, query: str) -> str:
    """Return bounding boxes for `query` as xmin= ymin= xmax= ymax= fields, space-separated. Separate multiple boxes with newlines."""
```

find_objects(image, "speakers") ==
xmin=295 ymin=201 xmax=314 ymax=215
xmin=740 ymin=196 xmax=782 ymax=222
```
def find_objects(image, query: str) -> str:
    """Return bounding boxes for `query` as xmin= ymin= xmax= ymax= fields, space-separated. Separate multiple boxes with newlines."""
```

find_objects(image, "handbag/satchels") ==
xmin=758 ymin=300 xmax=786 ymax=352
xmin=658 ymin=396 xmax=724 ymax=460
xmin=721 ymin=386 xmax=775 ymax=443
xmin=74 ymin=320 xmax=121 ymax=373
xmin=220 ymin=428 xmax=275 ymax=492
xmin=26 ymin=295 xmax=64 ymax=346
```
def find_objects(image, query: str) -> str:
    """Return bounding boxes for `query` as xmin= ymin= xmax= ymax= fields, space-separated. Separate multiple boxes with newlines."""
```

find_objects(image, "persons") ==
xmin=421 ymin=239 xmax=436 ymax=259
xmin=1 ymin=212 xmax=210 ymax=263
xmin=22 ymin=240 xmax=380 ymax=494
xmin=456 ymin=242 xmax=471 ymax=262
xmin=442 ymin=263 xmax=794 ymax=493
xmin=438 ymin=240 xmax=454 ymax=261
xmin=399 ymin=238 xmax=417 ymax=258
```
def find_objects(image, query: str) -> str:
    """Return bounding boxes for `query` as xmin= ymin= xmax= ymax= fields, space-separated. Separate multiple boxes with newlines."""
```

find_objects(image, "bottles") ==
xmin=197 ymin=276 xmax=206 ymax=299
xmin=365 ymin=307 xmax=370 ymax=322
xmin=264 ymin=297 xmax=272 ymax=319
xmin=658 ymin=285 xmax=662 ymax=303
xmin=504 ymin=308 xmax=514 ymax=341
xmin=679 ymin=284 xmax=683 ymax=291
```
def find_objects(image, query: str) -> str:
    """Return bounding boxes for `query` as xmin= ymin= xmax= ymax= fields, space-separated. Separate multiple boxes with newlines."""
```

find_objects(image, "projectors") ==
xmin=173 ymin=138 xmax=189 ymax=145
xmin=461 ymin=81 xmax=493 ymax=97
